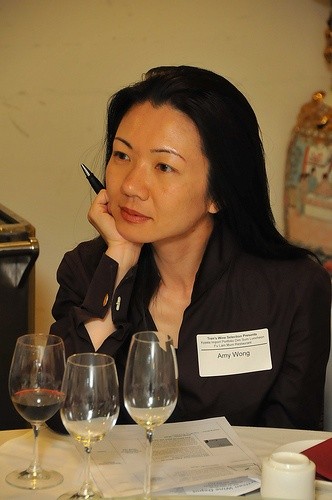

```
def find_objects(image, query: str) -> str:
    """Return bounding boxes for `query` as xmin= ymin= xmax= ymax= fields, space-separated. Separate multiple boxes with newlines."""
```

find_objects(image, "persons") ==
xmin=33 ymin=65 xmax=332 ymax=435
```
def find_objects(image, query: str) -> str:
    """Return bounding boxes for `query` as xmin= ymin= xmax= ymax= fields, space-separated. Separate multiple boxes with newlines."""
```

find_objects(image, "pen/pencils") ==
xmin=81 ymin=164 xmax=105 ymax=194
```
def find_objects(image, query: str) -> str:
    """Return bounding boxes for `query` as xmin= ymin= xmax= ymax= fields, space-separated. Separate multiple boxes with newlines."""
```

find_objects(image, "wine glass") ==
xmin=125 ymin=332 xmax=179 ymax=500
xmin=4 ymin=333 xmax=67 ymax=490
xmin=56 ymin=354 xmax=121 ymax=500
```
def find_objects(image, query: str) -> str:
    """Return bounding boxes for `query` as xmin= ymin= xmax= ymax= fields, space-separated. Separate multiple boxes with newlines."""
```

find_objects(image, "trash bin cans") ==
xmin=0 ymin=204 xmax=39 ymax=430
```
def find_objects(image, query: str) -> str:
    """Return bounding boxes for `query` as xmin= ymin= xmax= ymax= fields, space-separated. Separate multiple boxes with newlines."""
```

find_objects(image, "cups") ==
xmin=260 ymin=451 xmax=317 ymax=500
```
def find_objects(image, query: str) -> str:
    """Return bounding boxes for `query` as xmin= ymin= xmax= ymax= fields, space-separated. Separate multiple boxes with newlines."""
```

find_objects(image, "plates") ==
xmin=273 ymin=440 xmax=332 ymax=487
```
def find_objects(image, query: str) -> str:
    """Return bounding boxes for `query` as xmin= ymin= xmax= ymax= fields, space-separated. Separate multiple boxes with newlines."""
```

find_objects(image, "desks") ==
xmin=0 ymin=426 xmax=331 ymax=499
